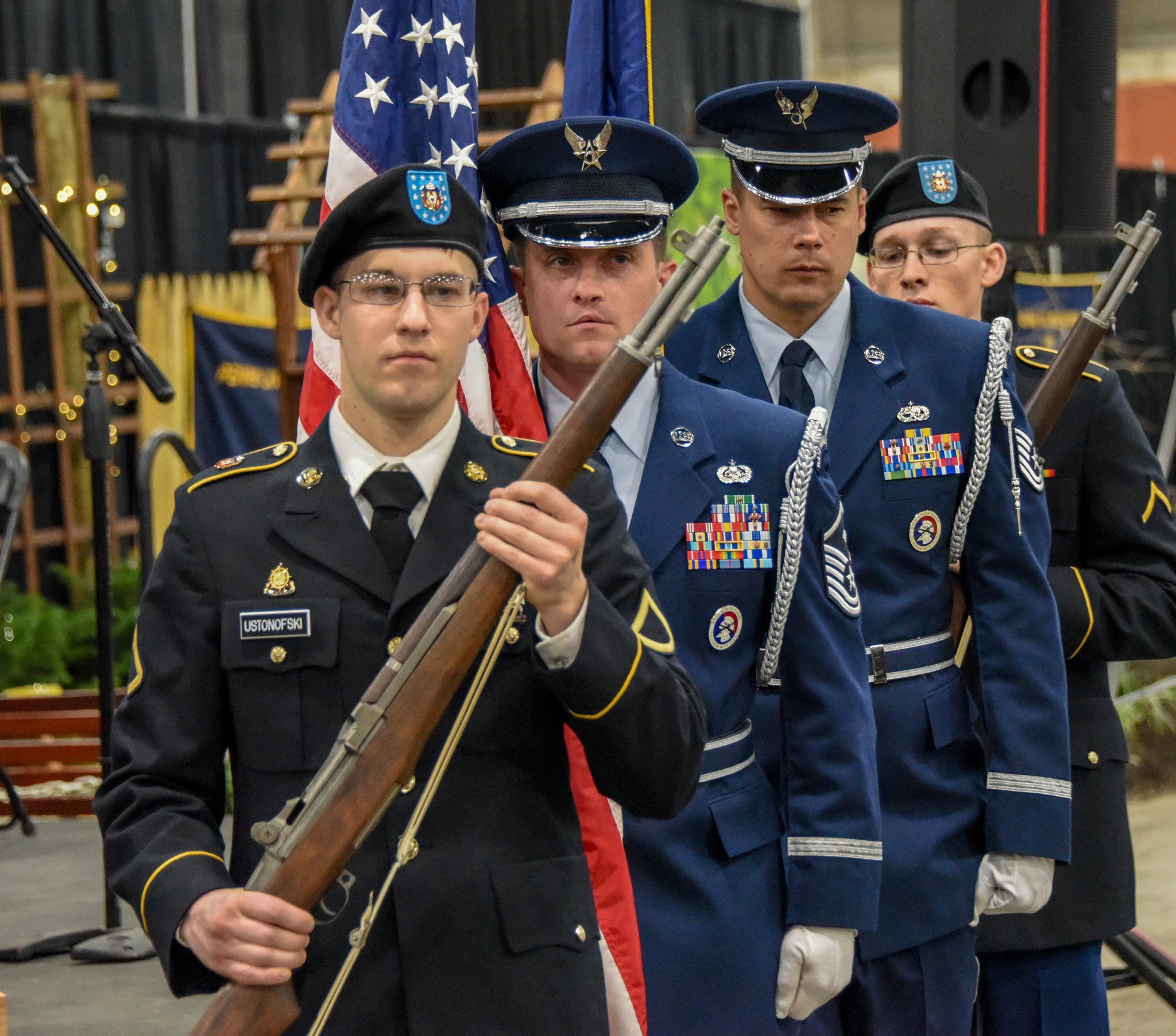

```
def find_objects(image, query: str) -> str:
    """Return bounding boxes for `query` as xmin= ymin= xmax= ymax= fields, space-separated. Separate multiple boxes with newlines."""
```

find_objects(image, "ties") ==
xmin=778 ymin=340 xmax=815 ymax=419
xmin=361 ymin=471 xmax=424 ymax=591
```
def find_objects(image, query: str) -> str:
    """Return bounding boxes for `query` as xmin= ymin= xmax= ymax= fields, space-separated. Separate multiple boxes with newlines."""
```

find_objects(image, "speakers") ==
xmin=890 ymin=3 xmax=1115 ymax=240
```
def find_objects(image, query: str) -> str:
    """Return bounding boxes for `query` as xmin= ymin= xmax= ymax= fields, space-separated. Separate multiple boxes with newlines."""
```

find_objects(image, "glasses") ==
xmin=334 ymin=272 xmax=483 ymax=307
xmin=866 ymin=244 xmax=990 ymax=269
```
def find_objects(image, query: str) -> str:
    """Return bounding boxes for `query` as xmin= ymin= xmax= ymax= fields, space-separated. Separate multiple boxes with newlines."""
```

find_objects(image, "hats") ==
xmin=297 ymin=164 xmax=486 ymax=309
xmin=695 ymin=80 xmax=900 ymax=208
xmin=478 ymin=115 xmax=699 ymax=248
xmin=856 ymin=154 xmax=992 ymax=257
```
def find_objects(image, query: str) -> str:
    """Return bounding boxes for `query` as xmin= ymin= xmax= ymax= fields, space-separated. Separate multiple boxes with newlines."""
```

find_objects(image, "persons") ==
xmin=476 ymin=111 xmax=887 ymax=1036
xmin=90 ymin=159 xmax=705 ymax=1036
xmin=664 ymin=72 xmax=1079 ymax=1036
xmin=861 ymin=154 xmax=1175 ymax=1036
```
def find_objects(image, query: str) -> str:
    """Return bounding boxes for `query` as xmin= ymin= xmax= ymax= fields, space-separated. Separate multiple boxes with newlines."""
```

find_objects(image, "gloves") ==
xmin=775 ymin=924 xmax=856 ymax=1021
xmin=968 ymin=853 xmax=1054 ymax=927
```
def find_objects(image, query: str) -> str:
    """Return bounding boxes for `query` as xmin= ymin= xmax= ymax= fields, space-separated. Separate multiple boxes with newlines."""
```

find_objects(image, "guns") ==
xmin=1014 ymin=202 xmax=1159 ymax=456
xmin=174 ymin=212 xmax=739 ymax=1036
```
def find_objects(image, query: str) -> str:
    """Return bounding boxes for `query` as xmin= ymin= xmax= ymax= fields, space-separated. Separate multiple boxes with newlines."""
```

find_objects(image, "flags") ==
xmin=292 ymin=2 xmax=651 ymax=1033
xmin=562 ymin=0 xmax=648 ymax=120
